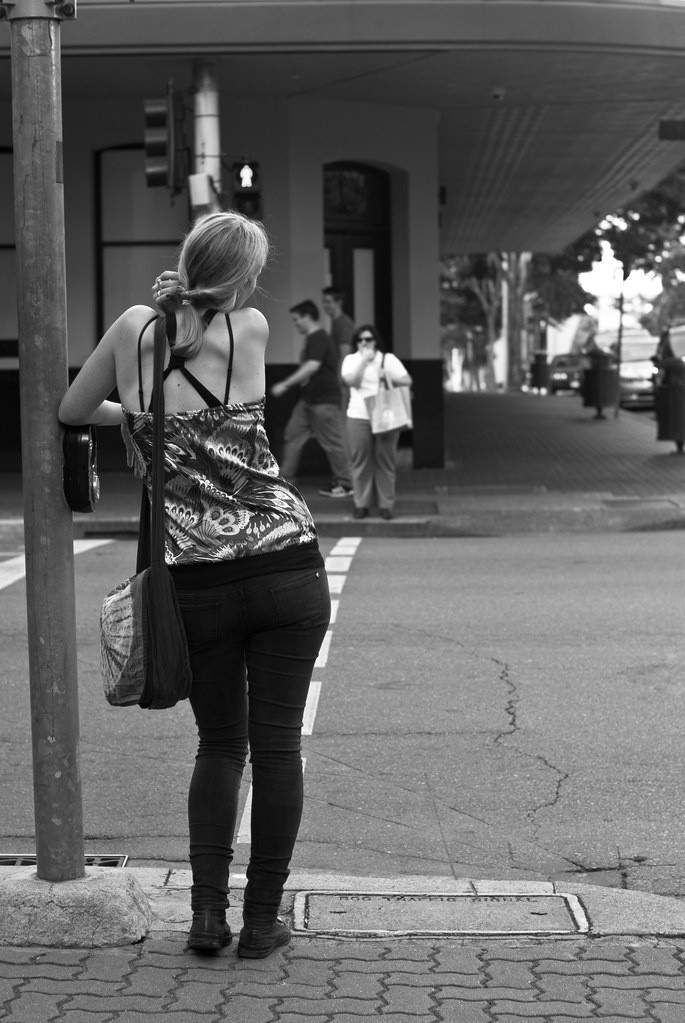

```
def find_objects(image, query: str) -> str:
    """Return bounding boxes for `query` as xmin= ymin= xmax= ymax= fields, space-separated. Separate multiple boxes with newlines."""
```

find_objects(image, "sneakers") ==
xmin=317 ymin=484 xmax=354 ymax=498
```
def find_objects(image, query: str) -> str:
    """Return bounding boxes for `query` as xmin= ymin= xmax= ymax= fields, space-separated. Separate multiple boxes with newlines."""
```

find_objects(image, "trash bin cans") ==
xmin=582 ymin=348 xmax=620 ymax=407
xmin=531 ymin=353 xmax=550 ymax=388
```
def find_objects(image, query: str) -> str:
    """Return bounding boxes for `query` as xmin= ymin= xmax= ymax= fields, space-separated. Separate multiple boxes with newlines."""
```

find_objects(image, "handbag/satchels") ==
xmin=360 ymin=375 xmax=413 ymax=435
xmin=99 ymin=565 xmax=190 ymax=711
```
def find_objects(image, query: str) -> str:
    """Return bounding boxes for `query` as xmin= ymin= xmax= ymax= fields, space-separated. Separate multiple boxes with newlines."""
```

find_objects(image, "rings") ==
xmin=158 ymin=278 xmax=162 ymax=290
xmin=158 ymin=290 xmax=161 ymax=297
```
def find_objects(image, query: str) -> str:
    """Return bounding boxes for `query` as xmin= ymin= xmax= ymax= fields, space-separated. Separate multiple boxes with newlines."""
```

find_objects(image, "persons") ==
xmin=273 ymin=300 xmax=353 ymax=497
xmin=58 ymin=212 xmax=332 ymax=960
xmin=340 ymin=324 xmax=413 ymax=520
xmin=322 ymin=286 xmax=355 ymax=358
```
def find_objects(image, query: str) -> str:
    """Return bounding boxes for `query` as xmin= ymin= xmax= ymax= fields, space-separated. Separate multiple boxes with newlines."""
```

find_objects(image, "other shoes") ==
xmin=189 ymin=914 xmax=231 ymax=949
xmin=237 ymin=920 xmax=290 ymax=959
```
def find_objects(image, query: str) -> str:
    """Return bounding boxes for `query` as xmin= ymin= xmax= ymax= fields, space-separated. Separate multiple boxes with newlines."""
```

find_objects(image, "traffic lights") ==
xmin=231 ymin=161 xmax=264 ymax=221
xmin=142 ymin=91 xmax=174 ymax=188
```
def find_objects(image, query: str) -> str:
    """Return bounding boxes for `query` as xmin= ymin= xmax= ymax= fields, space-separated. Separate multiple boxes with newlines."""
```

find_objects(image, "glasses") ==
xmin=358 ymin=337 xmax=373 ymax=342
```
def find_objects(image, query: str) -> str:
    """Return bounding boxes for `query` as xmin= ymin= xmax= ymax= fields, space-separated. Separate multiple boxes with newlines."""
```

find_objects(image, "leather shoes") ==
xmin=380 ymin=508 xmax=393 ymax=520
xmin=353 ymin=505 xmax=370 ymax=519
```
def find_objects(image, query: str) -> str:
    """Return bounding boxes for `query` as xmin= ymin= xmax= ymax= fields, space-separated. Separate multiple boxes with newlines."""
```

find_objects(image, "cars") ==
xmin=600 ymin=326 xmax=661 ymax=410
xmin=653 ymin=325 xmax=685 ymax=396
xmin=551 ymin=351 xmax=597 ymax=396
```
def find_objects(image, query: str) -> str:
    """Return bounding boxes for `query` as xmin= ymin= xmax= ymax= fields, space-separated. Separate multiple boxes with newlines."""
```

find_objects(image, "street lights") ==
xmin=612 ymin=291 xmax=627 ymax=420
